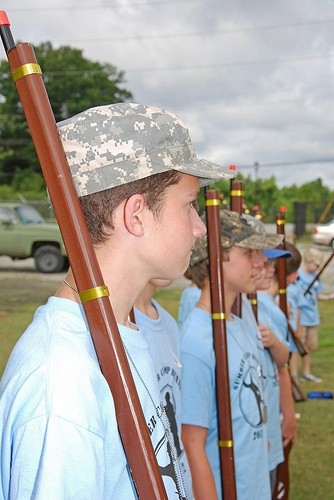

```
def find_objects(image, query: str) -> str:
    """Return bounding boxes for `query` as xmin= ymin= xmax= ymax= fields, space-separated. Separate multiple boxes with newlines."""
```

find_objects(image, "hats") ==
xmin=241 ymin=214 xmax=265 ymax=233
xmin=302 ymin=248 xmax=325 ymax=267
xmin=263 ymin=248 xmax=292 ymax=260
xmin=46 ymin=103 xmax=239 ymax=207
xmin=188 ymin=210 xmax=285 ymax=268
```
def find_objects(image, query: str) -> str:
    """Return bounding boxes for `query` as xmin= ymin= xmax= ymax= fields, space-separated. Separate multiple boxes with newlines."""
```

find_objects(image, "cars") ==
xmin=313 ymin=220 xmax=334 ymax=249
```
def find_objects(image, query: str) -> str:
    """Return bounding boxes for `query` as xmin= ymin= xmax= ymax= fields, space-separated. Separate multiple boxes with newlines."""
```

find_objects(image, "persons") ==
xmin=0 ymin=102 xmax=238 ymax=499
xmin=129 ymin=278 xmax=193 ymax=500
xmin=180 ymin=239 xmax=334 ymax=500
xmin=180 ymin=209 xmax=284 ymax=500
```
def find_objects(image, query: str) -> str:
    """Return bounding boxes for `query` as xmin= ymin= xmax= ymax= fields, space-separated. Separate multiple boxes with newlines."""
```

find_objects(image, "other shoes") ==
xmin=304 ymin=374 xmax=322 ymax=383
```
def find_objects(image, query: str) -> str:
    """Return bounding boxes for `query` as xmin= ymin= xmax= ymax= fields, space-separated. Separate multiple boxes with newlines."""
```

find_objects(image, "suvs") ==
xmin=0 ymin=203 xmax=70 ymax=274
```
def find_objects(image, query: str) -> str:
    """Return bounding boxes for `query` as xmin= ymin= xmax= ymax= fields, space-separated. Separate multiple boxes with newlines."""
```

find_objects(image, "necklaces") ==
xmin=62 ymin=280 xmax=188 ymax=499
xmin=202 ymin=300 xmax=263 ymax=380
